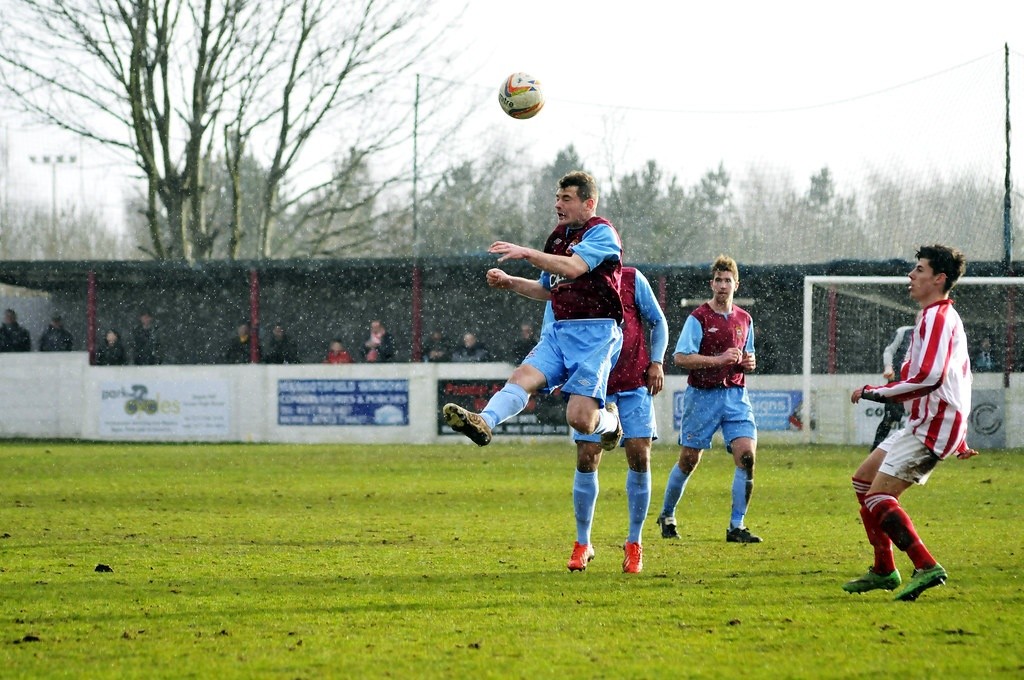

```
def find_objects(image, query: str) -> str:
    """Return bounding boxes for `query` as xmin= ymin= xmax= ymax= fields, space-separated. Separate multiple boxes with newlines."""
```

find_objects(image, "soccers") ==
xmin=498 ymin=72 xmax=546 ymax=120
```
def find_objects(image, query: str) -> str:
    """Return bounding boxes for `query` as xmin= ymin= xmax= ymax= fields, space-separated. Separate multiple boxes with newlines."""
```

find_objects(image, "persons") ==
xmin=225 ymin=321 xmax=250 ymax=363
xmin=358 ymin=322 xmax=398 ymax=364
xmin=260 ymin=323 xmax=300 ymax=364
xmin=0 ymin=308 xmax=32 ymax=351
xmin=421 ymin=331 xmax=449 ymax=361
xmin=973 ymin=336 xmax=1002 ymax=372
xmin=507 ymin=325 xmax=537 ymax=366
xmin=131 ymin=309 xmax=162 ymax=365
xmin=869 ymin=310 xmax=924 ymax=455
xmin=657 ymin=256 xmax=759 ymax=543
xmin=537 ymin=267 xmax=670 ymax=574
xmin=451 ymin=331 xmax=496 ymax=362
xmin=843 ymin=244 xmax=981 ymax=601
xmin=95 ymin=329 xmax=127 ymax=365
xmin=441 ymin=171 xmax=626 ymax=452
xmin=322 ymin=339 xmax=354 ymax=364
xmin=37 ymin=312 xmax=73 ymax=352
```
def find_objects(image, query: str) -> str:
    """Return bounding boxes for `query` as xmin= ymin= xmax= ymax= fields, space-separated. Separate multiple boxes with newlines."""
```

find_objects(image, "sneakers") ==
xmin=841 ymin=565 xmax=902 ymax=594
xmin=727 ymin=527 xmax=764 ymax=543
xmin=623 ymin=540 xmax=643 ymax=574
xmin=443 ymin=402 xmax=493 ymax=447
xmin=567 ymin=541 xmax=595 ymax=572
xmin=895 ymin=561 xmax=948 ymax=601
xmin=600 ymin=402 xmax=622 ymax=452
xmin=657 ymin=513 xmax=681 ymax=541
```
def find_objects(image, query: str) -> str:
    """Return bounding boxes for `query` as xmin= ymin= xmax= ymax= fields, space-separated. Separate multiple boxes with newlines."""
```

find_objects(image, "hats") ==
xmin=52 ymin=316 xmax=62 ymax=321
